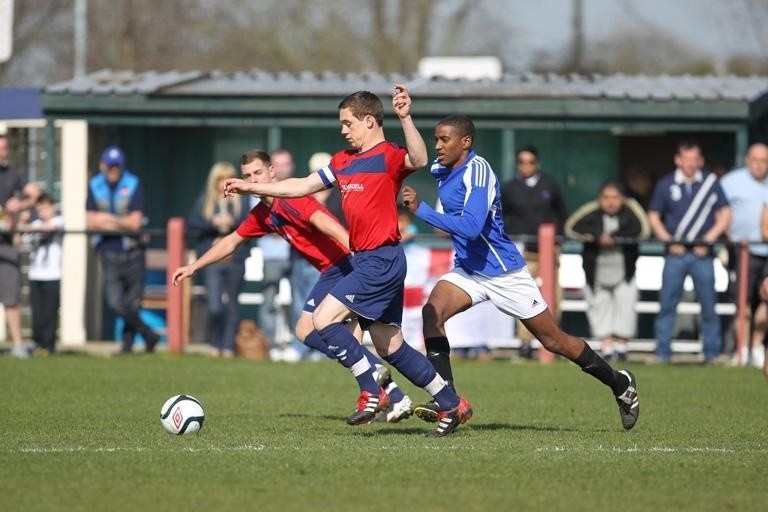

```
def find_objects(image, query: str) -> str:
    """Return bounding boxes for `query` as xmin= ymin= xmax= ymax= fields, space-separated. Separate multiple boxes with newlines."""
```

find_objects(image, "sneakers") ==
xmin=729 ymin=344 xmax=767 ymax=368
xmin=346 ymin=386 xmax=389 ymax=425
xmin=414 ymin=399 xmax=439 ymax=422
xmin=429 ymin=397 xmax=474 ymax=438
xmin=615 ymin=369 xmax=639 ymax=430
xmin=144 ymin=335 xmax=159 ymax=352
xmin=386 ymin=395 xmax=414 ymax=423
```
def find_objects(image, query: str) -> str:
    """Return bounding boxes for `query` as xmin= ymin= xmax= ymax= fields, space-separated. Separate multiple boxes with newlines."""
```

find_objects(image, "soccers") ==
xmin=160 ymin=395 xmax=205 ymax=434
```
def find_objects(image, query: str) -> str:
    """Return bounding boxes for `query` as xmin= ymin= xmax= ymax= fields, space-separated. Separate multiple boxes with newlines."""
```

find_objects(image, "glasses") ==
xmin=518 ymin=159 xmax=537 ymax=165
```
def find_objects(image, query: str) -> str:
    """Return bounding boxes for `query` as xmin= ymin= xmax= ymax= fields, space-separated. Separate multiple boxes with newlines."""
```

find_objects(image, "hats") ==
xmin=99 ymin=145 xmax=126 ymax=168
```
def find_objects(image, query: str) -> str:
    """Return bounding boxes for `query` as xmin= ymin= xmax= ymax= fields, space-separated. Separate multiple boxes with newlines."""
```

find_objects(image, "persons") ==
xmin=499 ymin=144 xmax=766 ymax=369
xmin=1 ymin=138 xmax=162 ymax=354
xmin=171 ymin=84 xmax=639 ymax=434
